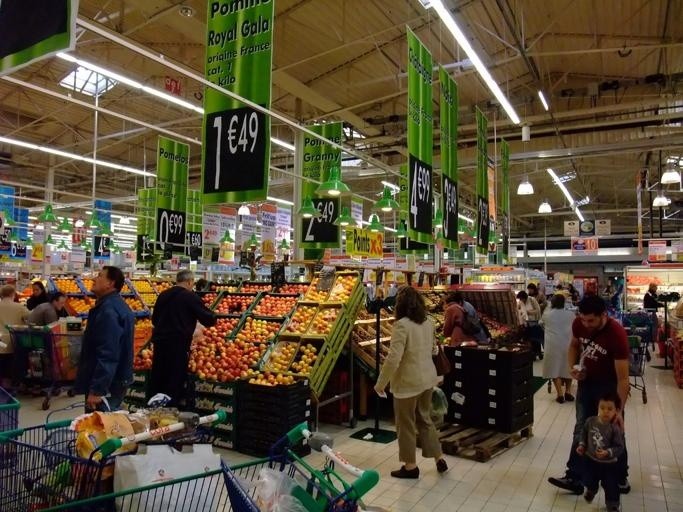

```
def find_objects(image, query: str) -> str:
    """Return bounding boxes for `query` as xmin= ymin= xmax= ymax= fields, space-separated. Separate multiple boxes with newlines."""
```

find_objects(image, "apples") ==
xmin=133 ymin=272 xmax=357 ymax=390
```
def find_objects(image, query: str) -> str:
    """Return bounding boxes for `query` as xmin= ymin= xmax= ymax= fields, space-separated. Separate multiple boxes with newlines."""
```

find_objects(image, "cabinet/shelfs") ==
xmin=46 ymin=273 xmax=511 ymax=397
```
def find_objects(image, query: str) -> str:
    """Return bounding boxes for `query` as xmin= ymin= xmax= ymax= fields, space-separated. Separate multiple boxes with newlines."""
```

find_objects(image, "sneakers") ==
xmin=556 ymin=396 xmax=564 ymax=403
xmin=548 ymin=477 xmax=584 ymax=494
xmin=565 ymin=393 xmax=574 ymax=401
xmin=618 ymin=478 xmax=630 ymax=494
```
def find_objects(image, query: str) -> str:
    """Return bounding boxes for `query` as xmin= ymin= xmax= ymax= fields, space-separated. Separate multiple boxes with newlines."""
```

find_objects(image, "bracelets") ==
xmin=567 ymin=366 xmax=572 ymax=370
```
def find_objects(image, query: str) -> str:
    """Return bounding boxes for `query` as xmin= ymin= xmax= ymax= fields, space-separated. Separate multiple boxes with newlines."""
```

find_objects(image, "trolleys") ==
xmin=0 ymin=404 xmax=227 ymax=512
xmin=620 ymin=332 xmax=648 ymax=404
xmin=546 ymin=346 xmax=580 ymax=393
xmin=0 ymin=386 xmax=22 ymax=469
xmin=34 ymin=420 xmax=380 ymax=511
xmin=619 ymin=306 xmax=655 ymax=363
xmin=5 ymin=322 xmax=82 ymax=411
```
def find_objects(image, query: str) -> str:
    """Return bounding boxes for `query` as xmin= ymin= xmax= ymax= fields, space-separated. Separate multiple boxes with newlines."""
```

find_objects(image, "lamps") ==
xmin=429 ymin=0 xmax=520 ymax=125
xmin=3 ymin=158 xmax=496 ymax=251
xmin=652 ymin=184 xmax=671 ymax=206
xmin=516 ymin=175 xmax=534 ymax=195
xmin=537 ymin=198 xmax=552 ymax=214
xmin=661 ymin=163 xmax=681 ymax=184
xmin=546 ymin=167 xmax=584 ymax=222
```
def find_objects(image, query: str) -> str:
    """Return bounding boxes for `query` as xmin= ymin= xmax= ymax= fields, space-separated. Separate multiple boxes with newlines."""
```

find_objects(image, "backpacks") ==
xmin=457 ymin=306 xmax=480 ymax=335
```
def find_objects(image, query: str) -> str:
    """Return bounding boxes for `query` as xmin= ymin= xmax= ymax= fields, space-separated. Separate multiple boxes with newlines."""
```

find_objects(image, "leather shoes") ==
xmin=436 ymin=459 xmax=447 ymax=473
xmin=391 ymin=465 xmax=419 ymax=478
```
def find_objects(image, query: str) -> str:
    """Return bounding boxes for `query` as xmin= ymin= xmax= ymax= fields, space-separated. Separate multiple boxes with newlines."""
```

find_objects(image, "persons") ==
xmin=373 ymin=285 xmax=448 ymax=479
xmin=0 ymin=285 xmax=29 ymax=390
xmin=25 ymin=281 xmax=47 ymax=311
xmin=575 ymin=391 xmax=625 ymax=511
xmin=16 ymin=292 xmax=67 ymax=396
xmin=75 ymin=266 xmax=134 ymax=416
xmin=195 ymin=278 xmax=207 ymax=292
xmin=429 ymin=282 xmax=664 ymax=403
xmin=146 ymin=269 xmax=217 ymax=452
xmin=547 ymin=294 xmax=630 ymax=494
